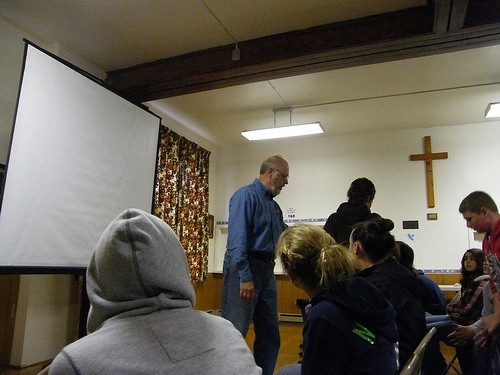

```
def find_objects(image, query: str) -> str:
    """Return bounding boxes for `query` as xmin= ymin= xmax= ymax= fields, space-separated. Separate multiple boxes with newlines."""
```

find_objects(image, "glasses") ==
xmin=271 ymin=169 xmax=289 ymax=181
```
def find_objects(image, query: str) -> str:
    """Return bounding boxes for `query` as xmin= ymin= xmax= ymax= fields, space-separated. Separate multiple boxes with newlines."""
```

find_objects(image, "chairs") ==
xmin=394 ymin=327 xmax=462 ymax=375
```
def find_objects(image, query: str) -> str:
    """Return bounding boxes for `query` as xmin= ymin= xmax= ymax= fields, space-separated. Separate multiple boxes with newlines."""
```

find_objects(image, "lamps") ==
xmin=484 ymin=103 xmax=500 ymax=118
xmin=240 ymin=107 xmax=326 ymax=142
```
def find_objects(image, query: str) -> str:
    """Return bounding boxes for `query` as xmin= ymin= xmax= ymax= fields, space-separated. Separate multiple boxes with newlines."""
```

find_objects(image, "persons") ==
xmin=425 ymin=248 xmax=484 ymax=375
xmin=221 ymin=155 xmax=289 ymax=375
xmin=275 ymin=218 xmax=500 ymax=375
xmin=323 ymin=177 xmax=376 ymax=249
xmin=447 ymin=190 xmax=500 ymax=375
xmin=277 ymin=223 xmax=399 ymax=375
xmin=48 ymin=208 xmax=263 ymax=375
